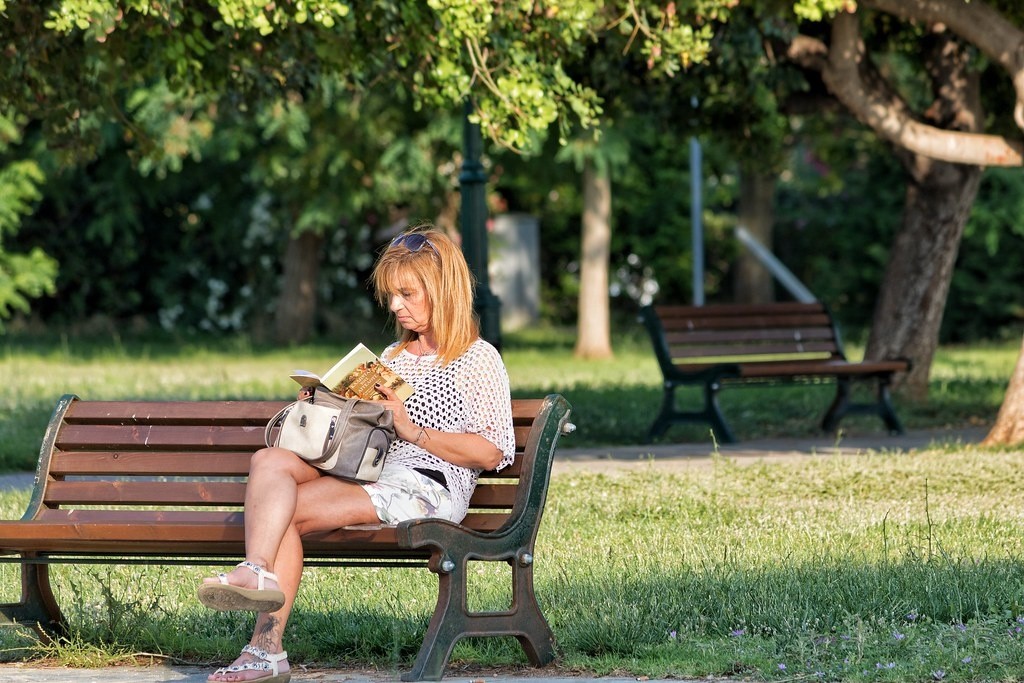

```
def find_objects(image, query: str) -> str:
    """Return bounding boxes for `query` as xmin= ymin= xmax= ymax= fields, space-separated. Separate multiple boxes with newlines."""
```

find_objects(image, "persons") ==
xmin=200 ymin=224 xmax=516 ymax=683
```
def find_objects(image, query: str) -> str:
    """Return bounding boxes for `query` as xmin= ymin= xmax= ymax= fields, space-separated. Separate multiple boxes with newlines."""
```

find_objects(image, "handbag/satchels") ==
xmin=264 ymin=384 xmax=397 ymax=484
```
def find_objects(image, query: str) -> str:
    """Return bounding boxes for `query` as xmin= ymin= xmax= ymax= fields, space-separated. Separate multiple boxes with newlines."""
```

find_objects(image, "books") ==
xmin=289 ymin=342 xmax=415 ymax=404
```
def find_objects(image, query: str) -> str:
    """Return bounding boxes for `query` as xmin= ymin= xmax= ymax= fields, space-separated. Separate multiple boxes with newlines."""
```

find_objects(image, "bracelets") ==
xmin=416 ymin=426 xmax=427 ymax=444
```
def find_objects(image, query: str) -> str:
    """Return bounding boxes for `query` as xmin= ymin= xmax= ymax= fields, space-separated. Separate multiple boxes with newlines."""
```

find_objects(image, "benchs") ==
xmin=636 ymin=300 xmax=915 ymax=443
xmin=1 ymin=390 xmax=575 ymax=683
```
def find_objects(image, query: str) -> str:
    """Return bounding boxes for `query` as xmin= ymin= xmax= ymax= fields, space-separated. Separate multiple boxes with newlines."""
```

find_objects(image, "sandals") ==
xmin=196 ymin=562 xmax=285 ymax=613
xmin=207 ymin=644 xmax=290 ymax=683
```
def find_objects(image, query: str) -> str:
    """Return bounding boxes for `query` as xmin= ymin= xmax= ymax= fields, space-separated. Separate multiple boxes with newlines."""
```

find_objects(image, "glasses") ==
xmin=389 ymin=234 xmax=440 ymax=258
